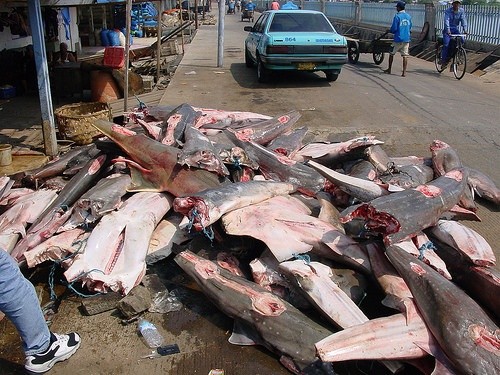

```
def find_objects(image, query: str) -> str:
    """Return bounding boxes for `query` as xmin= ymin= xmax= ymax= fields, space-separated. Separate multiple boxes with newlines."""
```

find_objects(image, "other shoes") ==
xmin=441 ymin=64 xmax=447 ymax=70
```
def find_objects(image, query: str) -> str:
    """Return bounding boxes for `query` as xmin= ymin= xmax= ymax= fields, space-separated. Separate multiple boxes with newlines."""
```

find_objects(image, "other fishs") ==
xmin=0 ymin=93 xmax=499 ymax=375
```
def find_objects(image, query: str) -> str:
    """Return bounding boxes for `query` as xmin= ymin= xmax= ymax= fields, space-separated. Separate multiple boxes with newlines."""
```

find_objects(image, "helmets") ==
xmin=395 ymin=1 xmax=406 ymax=7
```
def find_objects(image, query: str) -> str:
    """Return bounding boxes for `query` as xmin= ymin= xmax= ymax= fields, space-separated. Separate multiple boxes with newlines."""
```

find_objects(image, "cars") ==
xmin=244 ymin=1 xmax=348 ymax=84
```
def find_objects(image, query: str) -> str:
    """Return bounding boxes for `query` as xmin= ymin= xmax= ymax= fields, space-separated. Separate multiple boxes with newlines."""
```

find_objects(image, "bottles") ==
xmin=137 ymin=317 xmax=164 ymax=348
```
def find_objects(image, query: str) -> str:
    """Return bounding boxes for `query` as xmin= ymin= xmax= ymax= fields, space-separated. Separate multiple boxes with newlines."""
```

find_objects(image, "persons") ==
xmin=52 ymin=42 xmax=76 ymax=63
xmin=382 ymin=1 xmax=413 ymax=77
xmin=441 ymin=0 xmax=469 ymax=69
xmin=181 ymin=0 xmax=280 ymax=11
xmin=0 ymin=247 xmax=82 ymax=375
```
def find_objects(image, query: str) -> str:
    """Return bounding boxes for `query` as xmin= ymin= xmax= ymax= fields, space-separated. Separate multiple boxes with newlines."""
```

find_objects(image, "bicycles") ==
xmin=435 ymin=34 xmax=467 ymax=80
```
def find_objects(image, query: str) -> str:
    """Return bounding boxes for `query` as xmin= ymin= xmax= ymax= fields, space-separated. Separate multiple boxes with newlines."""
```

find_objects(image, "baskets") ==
xmin=56 ymin=101 xmax=114 ymax=146
xmin=104 ymin=48 xmax=125 ymax=69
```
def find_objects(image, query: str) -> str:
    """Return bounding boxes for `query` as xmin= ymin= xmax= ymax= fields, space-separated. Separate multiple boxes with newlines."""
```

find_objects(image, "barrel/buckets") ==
xmin=82 ymin=89 xmax=92 ymax=99
xmin=0 ymin=144 xmax=12 ymax=166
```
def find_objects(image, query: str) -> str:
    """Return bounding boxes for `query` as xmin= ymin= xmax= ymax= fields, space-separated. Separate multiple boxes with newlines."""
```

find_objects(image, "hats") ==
xmin=452 ymin=0 xmax=461 ymax=3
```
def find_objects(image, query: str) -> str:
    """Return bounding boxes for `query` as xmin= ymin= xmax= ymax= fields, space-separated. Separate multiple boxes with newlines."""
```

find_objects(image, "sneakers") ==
xmin=24 ymin=330 xmax=82 ymax=373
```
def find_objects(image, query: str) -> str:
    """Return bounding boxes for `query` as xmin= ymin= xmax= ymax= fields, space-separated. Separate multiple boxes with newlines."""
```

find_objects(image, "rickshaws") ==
xmin=241 ymin=9 xmax=250 ymax=22
xmin=345 ymin=30 xmax=394 ymax=65
